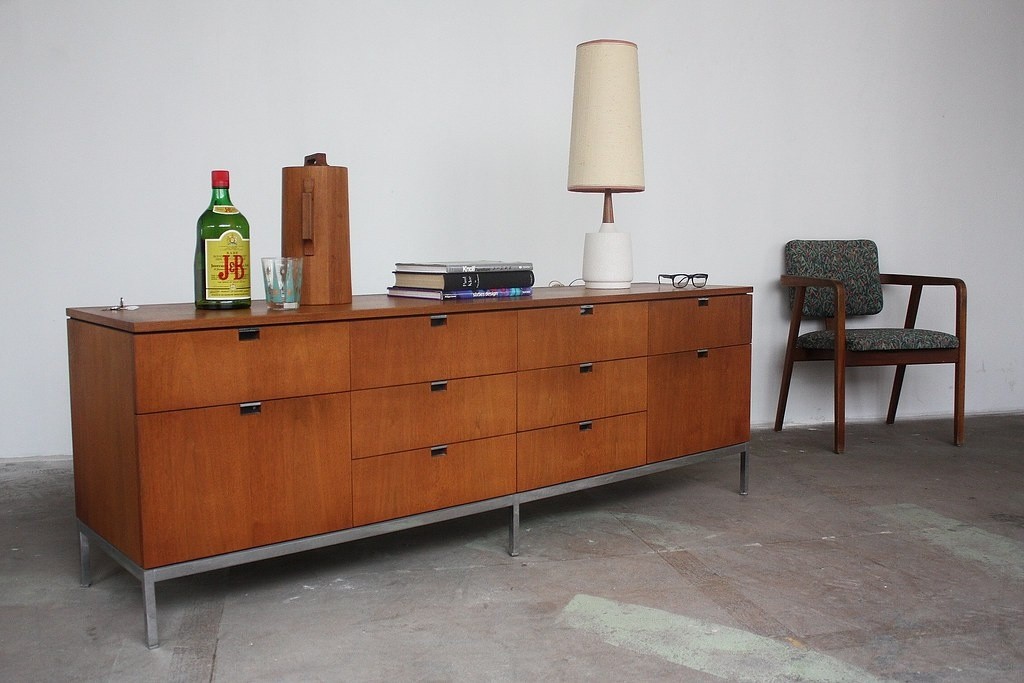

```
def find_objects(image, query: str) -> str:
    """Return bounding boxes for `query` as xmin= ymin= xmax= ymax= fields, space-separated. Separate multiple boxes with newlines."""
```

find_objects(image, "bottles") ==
xmin=194 ymin=171 xmax=252 ymax=309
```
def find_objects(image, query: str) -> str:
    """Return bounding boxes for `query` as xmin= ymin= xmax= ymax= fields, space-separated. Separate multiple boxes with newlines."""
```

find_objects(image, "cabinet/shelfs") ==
xmin=66 ymin=278 xmax=754 ymax=649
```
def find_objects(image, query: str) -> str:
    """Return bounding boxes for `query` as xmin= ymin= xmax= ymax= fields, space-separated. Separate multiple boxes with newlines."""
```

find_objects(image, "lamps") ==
xmin=568 ymin=39 xmax=645 ymax=289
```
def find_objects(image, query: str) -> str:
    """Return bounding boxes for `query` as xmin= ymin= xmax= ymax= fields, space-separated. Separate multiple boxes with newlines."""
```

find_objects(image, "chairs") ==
xmin=775 ymin=240 xmax=967 ymax=454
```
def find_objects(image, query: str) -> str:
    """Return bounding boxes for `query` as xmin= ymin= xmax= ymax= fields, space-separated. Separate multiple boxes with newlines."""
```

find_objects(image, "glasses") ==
xmin=658 ymin=273 xmax=709 ymax=288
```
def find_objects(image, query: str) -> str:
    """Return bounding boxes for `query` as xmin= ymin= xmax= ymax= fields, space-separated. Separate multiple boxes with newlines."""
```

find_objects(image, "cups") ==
xmin=262 ymin=257 xmax=303 ymax=311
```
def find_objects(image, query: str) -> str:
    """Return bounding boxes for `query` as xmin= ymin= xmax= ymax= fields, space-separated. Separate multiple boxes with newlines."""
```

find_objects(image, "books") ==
xmin=386 ymin=262 xmax=536 ymax=300
